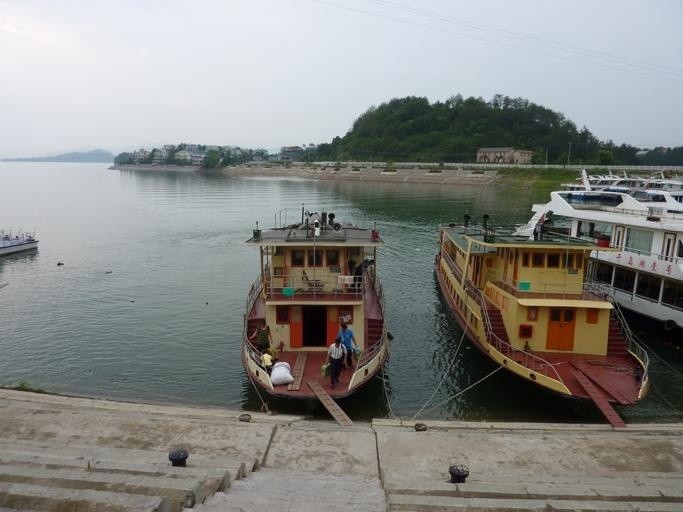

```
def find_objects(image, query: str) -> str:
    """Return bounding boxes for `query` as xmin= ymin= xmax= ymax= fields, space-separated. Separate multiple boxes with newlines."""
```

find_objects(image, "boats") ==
xmin=0 ymin=230 xmax=40 ymax=256
xmin=240 ymin=202 xmax=391 ymax=401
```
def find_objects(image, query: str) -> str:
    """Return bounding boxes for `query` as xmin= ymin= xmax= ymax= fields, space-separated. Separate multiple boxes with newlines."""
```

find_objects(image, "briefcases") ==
xmin=321 ymin=362 xmax=332 ymax=378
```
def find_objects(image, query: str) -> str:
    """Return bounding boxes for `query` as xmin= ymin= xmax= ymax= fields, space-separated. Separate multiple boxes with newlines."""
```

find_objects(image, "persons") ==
xmin=249 ymin=318 xmax=272 ymax=353
xmin=325 ymin=337 xmax=348 ymax=389
xmin=337 ymin=323 xmax=358 ymax=368
